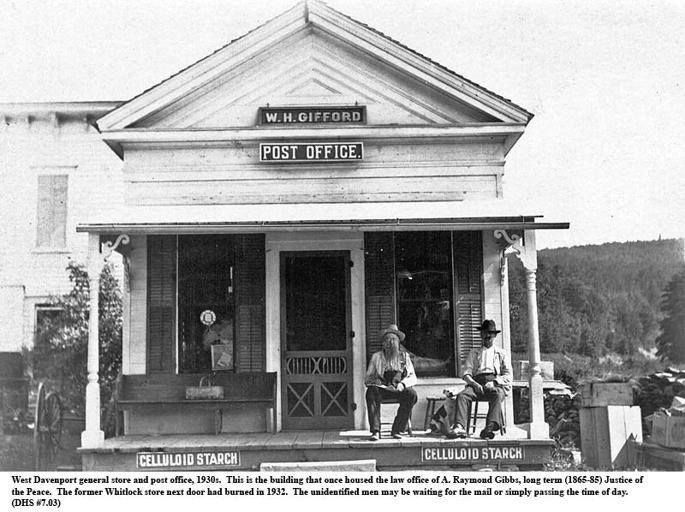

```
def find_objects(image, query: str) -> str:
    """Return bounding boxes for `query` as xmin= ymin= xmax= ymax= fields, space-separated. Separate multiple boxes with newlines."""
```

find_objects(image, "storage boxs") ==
xmin=578 ymin=406 xmax=642 ymax=469
xmin=581 ymin=382 xmax=633 ymax=408
xmin=652 ymin=411 xmax=685 ymax=449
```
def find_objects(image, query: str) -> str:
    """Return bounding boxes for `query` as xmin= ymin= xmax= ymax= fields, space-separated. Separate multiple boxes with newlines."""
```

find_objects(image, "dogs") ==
xmin=424 ymin=386 xmax=460 ymax=438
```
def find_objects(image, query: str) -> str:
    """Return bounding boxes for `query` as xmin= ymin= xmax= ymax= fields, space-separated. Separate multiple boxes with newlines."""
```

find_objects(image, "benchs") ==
xmin=116 ymin=373 xmax=280 ymax=432
xmin=630 ymin=441 xmax=685 ymax=471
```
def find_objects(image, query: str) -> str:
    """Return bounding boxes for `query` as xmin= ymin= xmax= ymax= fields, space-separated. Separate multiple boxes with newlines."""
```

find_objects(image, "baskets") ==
xmin=185 ymin=376 xmax=224 ymax=400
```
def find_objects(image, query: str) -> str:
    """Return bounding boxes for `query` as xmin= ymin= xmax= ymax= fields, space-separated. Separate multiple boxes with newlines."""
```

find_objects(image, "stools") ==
xmin=424 ymin=397 xmax=450 ymax=429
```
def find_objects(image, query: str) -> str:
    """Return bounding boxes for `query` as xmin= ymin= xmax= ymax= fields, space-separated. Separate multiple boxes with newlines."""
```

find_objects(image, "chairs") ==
xmin=379 ymin=397 xmax=412 ymax=437
xmin=466 ymin=384 xmax=507 ymax=436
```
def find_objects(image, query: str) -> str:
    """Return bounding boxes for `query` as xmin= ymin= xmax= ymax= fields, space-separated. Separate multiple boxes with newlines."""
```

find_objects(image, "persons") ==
xmin=444 ymin=318 xmax=513 ymax=440
xmin=362 ymin=322 xmax=419 ymax=441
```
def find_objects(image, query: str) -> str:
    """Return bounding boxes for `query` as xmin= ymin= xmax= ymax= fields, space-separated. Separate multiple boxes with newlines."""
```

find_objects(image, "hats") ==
xmin=378 ymin=324 xmax=406 ymax=342
xmin=476 ymin=320 xmax=501 ymax=333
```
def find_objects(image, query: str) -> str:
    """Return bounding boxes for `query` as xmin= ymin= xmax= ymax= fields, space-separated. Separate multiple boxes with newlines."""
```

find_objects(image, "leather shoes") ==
xmin=480 ymin=429 xmax=494 ymax=439
xmin=370 ymin=431 xmax=401 ymax=441
xmin=447 ymin=423 xmax=467 ymax=438
